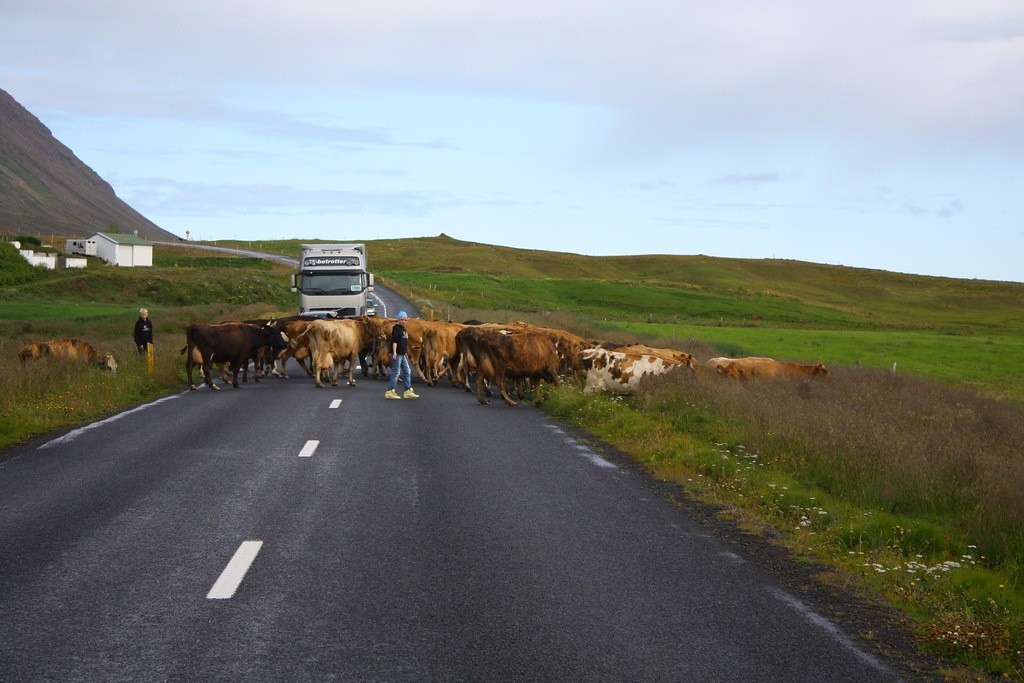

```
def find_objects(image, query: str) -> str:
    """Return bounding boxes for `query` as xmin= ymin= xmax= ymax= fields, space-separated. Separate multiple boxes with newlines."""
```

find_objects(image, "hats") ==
xmin=396 ymin=310 xmax=407 ymax=320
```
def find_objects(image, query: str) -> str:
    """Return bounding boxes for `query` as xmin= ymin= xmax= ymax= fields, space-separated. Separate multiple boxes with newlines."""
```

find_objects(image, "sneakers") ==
xmin=404 ymin=388 xmax=419 ymax=398
xmin=385 ymin=389 xmax=402 ymax=399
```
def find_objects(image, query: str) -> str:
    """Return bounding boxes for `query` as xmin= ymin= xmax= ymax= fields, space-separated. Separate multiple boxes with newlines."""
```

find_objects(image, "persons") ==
xmin=385 ymin=310 xmax=420 ymax=399
xmin=133 ymin=308 xmax=153 ymax=354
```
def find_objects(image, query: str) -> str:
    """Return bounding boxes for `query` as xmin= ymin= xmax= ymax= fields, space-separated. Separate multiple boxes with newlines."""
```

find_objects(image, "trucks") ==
xmin=288 ymin=242 xmax=375 ymax=320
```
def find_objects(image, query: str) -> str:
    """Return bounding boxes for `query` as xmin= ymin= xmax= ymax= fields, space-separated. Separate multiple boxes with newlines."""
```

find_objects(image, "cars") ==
xmin=366 ymin=299 xmax=378 ymax=316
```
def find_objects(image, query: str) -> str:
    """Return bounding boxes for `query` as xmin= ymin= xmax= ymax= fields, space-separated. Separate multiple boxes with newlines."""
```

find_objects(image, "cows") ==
xmin=176 ymin=313 xmax=832 ymax=409
xmin=17 ymin=337 xmax=120 ymax=379
